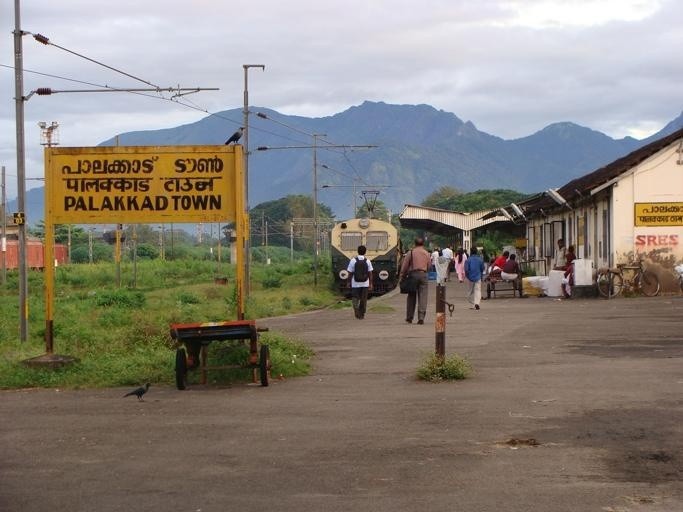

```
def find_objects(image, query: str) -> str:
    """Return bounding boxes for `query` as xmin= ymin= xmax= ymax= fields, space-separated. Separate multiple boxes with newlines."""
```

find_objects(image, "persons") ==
xmin=554 ymin=240 xmax=577 ymax=299
xmin=401 ymin=238 xmax=431 ymax=324
xmin=346 ymin=246 xmax=374 ymax=319
xmin=427 ymin=244 xmax=519 ymax=283
xmin=464 ymin=247 xmax=484 ymax=310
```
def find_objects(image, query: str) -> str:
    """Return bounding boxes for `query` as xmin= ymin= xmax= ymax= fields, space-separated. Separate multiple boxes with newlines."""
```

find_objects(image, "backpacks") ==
xmin=354 ymin=256 xmax=368 ymax=282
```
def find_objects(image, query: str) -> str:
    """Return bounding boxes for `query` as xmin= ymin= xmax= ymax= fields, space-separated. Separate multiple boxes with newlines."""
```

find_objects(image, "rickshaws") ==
xmin=485 ymin=260 xmax=522 ymax=298
xmin=168 ymin=320 xmax=270 ymax=388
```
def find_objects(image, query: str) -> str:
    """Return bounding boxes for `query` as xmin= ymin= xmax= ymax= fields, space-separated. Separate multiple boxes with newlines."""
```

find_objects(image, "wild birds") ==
xmin=225 ymin=127 xmax=245 ymax=145
xmin=122 ymin=382 xmax=152 ymax=400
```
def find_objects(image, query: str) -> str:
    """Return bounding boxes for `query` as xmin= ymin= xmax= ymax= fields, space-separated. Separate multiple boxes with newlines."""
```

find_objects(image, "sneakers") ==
xmin=405 ymin=318 xmax=413 ymax=323
xmin=475 ymin=304 xmax=480 ymax=309
xmin=417 ymin=320 xmax=424 ymax=324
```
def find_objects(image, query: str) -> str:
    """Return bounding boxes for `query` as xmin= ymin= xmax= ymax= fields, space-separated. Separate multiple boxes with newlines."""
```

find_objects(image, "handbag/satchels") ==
xmin=400 ymin=277 xmax=420 ymax=294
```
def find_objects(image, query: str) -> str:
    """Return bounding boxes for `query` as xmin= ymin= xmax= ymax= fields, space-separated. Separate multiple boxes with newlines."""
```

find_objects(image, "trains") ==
xmin=0 ymin=238 xmax=68 ymax=273
xmin=330 ymin=216 xmax=402 ymax=298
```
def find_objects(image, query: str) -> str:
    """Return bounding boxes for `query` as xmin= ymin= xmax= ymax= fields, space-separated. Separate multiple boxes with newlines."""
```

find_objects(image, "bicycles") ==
xmin=595 ymin=250 xmax=660 ymax=299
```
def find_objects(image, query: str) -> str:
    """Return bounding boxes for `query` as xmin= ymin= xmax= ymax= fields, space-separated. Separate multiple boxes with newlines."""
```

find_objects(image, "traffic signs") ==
xmin=634 ymin=201 xmax=683 ymax=227
